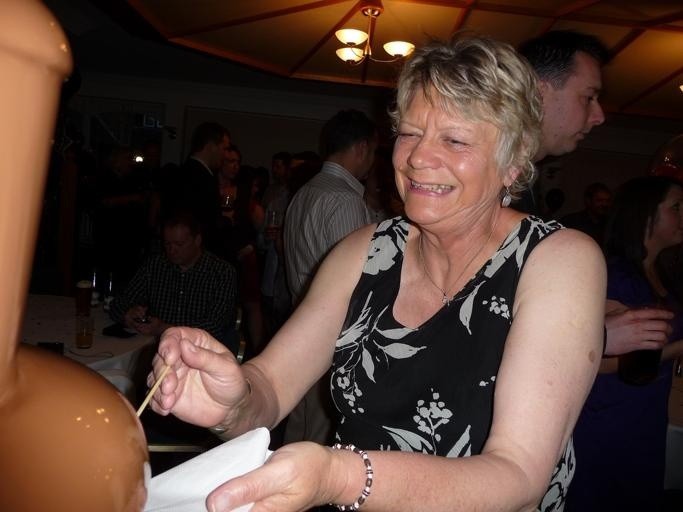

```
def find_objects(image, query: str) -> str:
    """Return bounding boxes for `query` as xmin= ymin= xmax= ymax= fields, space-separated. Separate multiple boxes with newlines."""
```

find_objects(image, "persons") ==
xmin=70 ymin=109 xmax=398 ymax=451
xmin=147 ymin=36 xmax=607 ymax=512
xmin=510 ymin=31 xmax=682 ymax=512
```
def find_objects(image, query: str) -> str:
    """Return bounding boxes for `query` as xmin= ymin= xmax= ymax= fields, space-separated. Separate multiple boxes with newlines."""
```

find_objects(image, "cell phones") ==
xmin=135 ymin=318 xmax=150 ymax=323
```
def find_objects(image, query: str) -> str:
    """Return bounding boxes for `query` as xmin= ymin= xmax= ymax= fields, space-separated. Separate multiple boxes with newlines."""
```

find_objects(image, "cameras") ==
xmin=37 ymin=342 xmax=64 ymax=355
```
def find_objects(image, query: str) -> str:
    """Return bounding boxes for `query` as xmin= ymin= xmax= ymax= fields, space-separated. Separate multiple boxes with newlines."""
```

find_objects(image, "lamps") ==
xmin=333 ymin=6 xmax=415 ymax=69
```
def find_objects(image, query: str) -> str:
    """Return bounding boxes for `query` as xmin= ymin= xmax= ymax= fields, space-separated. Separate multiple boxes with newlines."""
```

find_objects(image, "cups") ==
xmin=77 ymin=312 xmax=94 ymax=348
xmin=74 ymin=285 xmax=93 ymax=315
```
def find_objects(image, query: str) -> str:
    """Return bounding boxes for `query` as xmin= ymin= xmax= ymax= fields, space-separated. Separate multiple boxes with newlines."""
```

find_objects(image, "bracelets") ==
xmin=329 ymin=443 xmax=373 ymax=511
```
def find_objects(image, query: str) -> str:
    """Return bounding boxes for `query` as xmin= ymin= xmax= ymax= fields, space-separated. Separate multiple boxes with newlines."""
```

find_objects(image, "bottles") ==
xmin=103 ymin=280 xmax=115 ymax=313
xmin=90 ymin=272 xmax=100 ymax=308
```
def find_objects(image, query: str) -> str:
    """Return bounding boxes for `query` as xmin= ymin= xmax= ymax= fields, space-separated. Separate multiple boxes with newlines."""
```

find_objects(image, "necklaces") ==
xmin=418 ymin=212 xmax=499 ymax=303
